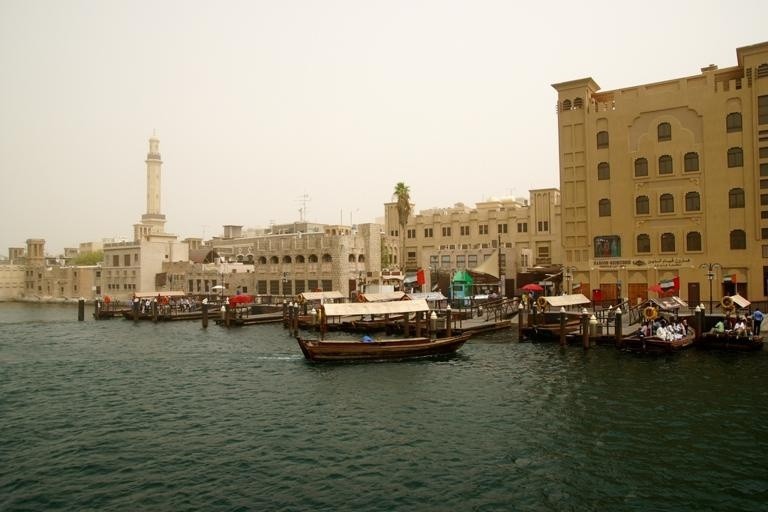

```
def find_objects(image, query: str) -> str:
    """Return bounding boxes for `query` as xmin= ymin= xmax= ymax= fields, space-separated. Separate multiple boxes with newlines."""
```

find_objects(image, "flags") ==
xmin=724 ymin=274 xmax=736 ymax=284
xmin=416 ymin=270 xmax=425 ymax=285
xmin=647 ymin=275 xmax=679 ymax=295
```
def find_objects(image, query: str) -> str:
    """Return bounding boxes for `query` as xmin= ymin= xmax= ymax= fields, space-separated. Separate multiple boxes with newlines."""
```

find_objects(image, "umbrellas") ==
xmin=521 ymin=284 xmax=543 ymax=291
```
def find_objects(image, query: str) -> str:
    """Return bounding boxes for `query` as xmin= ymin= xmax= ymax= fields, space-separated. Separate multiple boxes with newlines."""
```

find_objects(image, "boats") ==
xmin=294 ymin=331 xmax=472 ymax=362
xmin=624 ymin=319 xmax=696 ymax=349
xmin=702 ymin=323 xmax=764 ymax=349
xmin=519 ymin=311 xmax=591 ymax=338
xmin=121 ymin=305 xmax=253 ymax=321
xmin=286 ymin=310 xmax=427 ymax=327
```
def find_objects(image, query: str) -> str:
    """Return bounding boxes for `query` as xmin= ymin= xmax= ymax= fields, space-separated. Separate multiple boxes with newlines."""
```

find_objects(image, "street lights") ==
xmin=560 ymin=265 xmax=576 ymax=294
xmin=698 ymin=262 xmax=723 ymax=314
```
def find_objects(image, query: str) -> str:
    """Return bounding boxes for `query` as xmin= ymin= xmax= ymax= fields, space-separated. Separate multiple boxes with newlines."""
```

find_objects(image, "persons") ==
xmin=558 ymin=286 xmax=567 ymax=296
xmin=710 ymin=308 xmax=764 ymax=339
xmin=297 ymin=295 xmax=304 ymax=315
xmin=363 ymin=333 xmax=373 ymax=342
xmin=596 ymin=238 xmax=617 ymax=257
xmin=581 ymin=306 xmax=588 ymax=313
xmin=638 ymin=316 xmax=687 ymax=341
xmin=560 ymin=305 xmax=566 ymax=312
xmin=522 ymin=291 xmax=537 ymax=305
xmin=127 ymin=296 xmax=196 ymax=313
